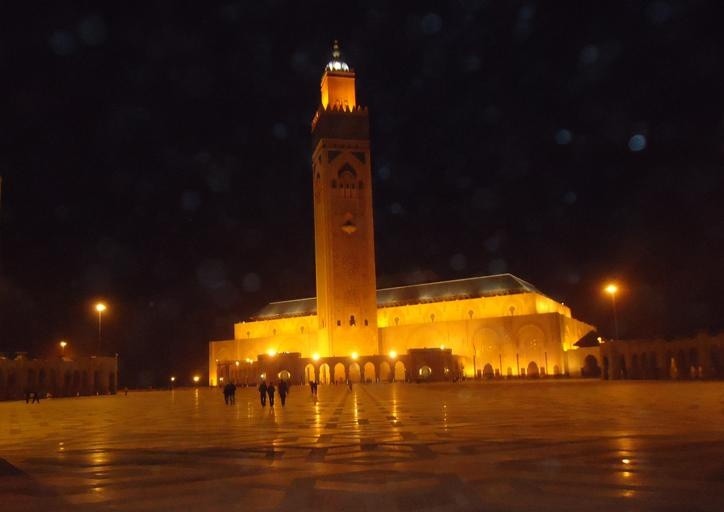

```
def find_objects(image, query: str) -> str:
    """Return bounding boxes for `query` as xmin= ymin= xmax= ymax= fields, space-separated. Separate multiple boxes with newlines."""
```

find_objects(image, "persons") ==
xmin=229 ymin=382 xmax=236 ymax=405
xmin=310 ymin=382 xmax=314 ymax=393
xmin=124 ymin=385 xmax=128 ymax=396
xmin=259 ymin=381 xmax=267 ymax=406
xmin=32 ymin=393 xmax=39 ymax=403
xmin=223 ymin=384 xmax=230 ymax=405
xmin=278 ymin=379 xmax=291 ymax=408
xmin=267 ymin=382 xmax=275 ymax=406
xmin=313 ymin=380 xmax=317 ymax=395
xmin=24 ymin=391 xmax=29 ymax=403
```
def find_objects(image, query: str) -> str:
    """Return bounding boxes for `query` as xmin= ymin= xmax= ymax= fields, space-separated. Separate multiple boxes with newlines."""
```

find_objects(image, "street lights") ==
xmin=96 ymin=304 xmax=109 ymax=343
xmin=59 ymin=341 xmax=66 ymax=353
xmin=606 ymin=285 xmax=620 ymax=339
